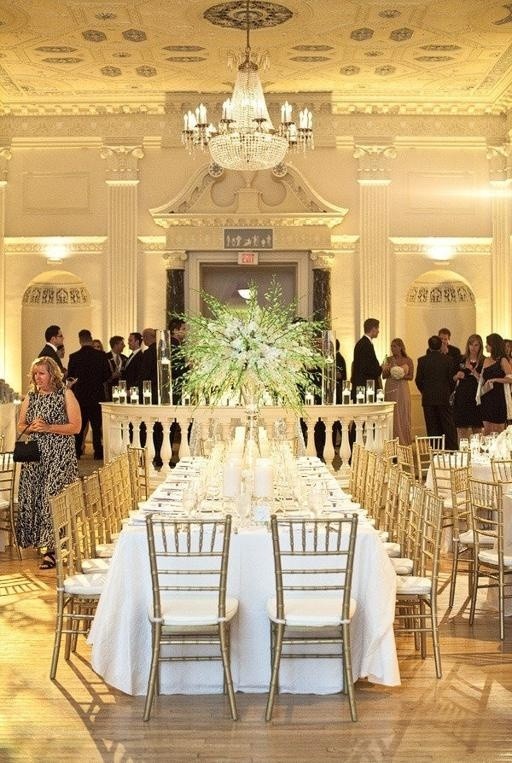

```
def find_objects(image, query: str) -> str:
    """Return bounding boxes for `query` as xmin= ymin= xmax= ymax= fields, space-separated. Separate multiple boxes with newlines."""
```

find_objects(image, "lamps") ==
xmin=182 ymin=3 xmax=316 ymax=174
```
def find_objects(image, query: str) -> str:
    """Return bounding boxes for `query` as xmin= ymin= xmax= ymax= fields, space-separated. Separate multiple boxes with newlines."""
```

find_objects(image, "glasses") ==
xmin=56 ymin=333 xmax=64 ymax=337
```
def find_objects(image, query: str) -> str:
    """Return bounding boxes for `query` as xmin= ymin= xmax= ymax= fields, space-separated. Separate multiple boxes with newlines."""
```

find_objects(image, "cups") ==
xmin=111 ymin=329 xmax=190 ymax=405
xmin=304 ymin=330 xmax=384 ymax=405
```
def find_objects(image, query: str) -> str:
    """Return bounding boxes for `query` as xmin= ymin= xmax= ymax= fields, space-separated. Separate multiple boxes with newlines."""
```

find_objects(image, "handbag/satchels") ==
xmin=479 ymin=379 xmax=494 ymax=397
xmin=11 ymin=422 xmax=41 ymax=465
xmin=447 ymin=374 xmax=460 ymax=408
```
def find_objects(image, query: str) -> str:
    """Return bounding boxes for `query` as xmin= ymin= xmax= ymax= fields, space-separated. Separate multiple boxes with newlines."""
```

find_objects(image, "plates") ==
xmin=125 ymin=457 xmax=370 ymax=529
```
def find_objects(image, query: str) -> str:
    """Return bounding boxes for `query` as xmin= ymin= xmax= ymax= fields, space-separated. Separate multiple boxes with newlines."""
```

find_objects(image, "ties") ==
xmin=124 ymin=352 xmax=133 ymax=368
xmin=116 ymin=354 xmax=122 ymax=369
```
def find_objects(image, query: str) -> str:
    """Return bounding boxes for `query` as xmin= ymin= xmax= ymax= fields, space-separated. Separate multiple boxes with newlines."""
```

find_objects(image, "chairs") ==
xmin=2 ymin=434 xmax=26 ymax=562
xmin=48 ymin=419 xmax=512 ymax=722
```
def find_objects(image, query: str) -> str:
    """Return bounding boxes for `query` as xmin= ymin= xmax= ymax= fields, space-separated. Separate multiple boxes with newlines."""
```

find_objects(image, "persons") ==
xmin=38 ymin=318 xmax=194 ymax=467
xmin=291 ymin=317 xmax=512 ymax=462
xmin=14 ymin=356 xmax=82 ymax=570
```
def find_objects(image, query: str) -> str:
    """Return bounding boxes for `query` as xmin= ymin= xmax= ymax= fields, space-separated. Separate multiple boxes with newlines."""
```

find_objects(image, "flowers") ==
xmin=159 ymin=271 xmax=344 ymax=419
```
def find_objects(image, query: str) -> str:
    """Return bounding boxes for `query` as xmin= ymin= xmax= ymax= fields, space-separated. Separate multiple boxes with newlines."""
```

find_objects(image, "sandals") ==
xmin=38 ymin=548 xmax=58 ymax=569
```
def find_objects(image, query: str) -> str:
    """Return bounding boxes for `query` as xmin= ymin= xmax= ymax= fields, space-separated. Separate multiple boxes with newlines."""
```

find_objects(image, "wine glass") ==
xmin=458 ymin=431 xmax=503 ymax=461
xmin=183 ymin=427 xmax=324 ymax=532
